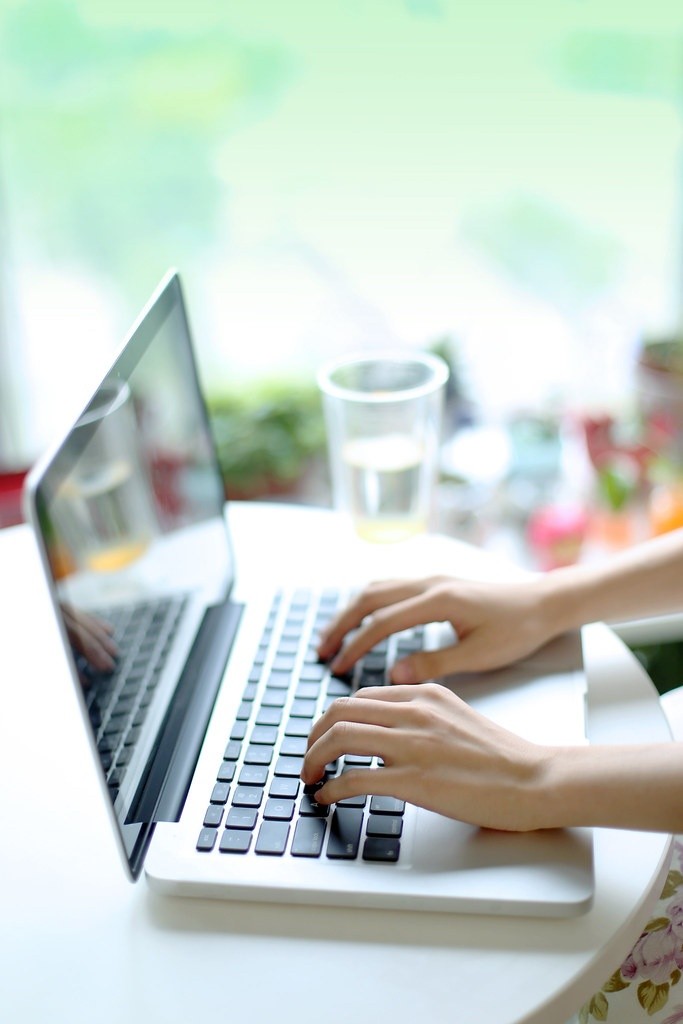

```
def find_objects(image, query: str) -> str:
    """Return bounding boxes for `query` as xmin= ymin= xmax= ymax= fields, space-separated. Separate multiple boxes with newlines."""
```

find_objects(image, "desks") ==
xmin=0 ymin=500 xmax=674 ymax=1024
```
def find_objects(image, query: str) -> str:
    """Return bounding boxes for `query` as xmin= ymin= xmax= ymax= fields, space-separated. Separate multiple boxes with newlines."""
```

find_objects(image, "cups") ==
xmin=317 ymin=350 xmax=450 ymax=552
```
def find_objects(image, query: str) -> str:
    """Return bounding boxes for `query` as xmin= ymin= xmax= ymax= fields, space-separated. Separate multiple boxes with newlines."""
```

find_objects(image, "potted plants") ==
xmin=198 ymin=372 xmax=326 ymax=500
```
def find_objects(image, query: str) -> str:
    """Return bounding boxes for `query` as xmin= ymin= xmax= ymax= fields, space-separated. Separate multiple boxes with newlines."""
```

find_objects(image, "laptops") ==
xmin=19 ymin=270 xmax=595 ymax=917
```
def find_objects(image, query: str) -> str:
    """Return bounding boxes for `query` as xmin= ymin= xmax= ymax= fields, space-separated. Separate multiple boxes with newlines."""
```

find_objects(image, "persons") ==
xmin=303 ymin=529 xmax=683 ymax=1024
xmin=58 ymin=597 xmax=122 ymax=673
